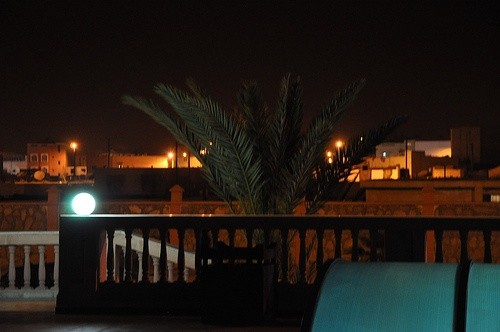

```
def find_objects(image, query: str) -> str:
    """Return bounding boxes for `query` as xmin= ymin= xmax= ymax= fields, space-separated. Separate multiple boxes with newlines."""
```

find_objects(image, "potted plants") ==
xmin=201 ymin=240 xmax=280 ymax=326
